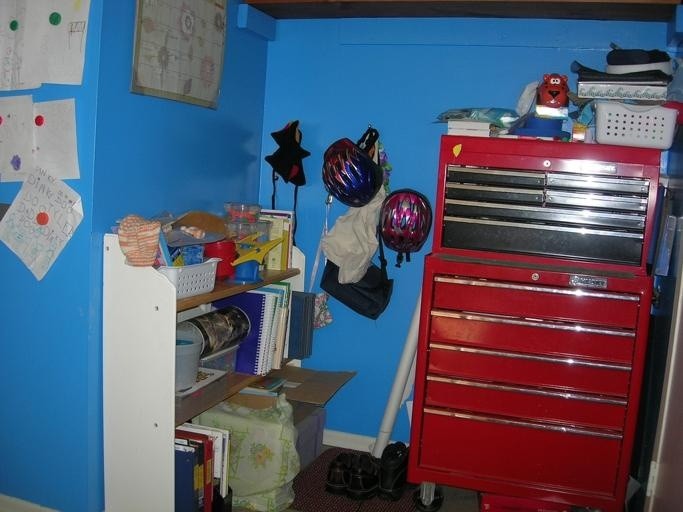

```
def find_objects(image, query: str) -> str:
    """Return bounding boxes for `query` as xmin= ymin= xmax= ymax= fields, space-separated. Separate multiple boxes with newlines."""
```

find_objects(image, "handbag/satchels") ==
xmin=319 ymin=259 xmax=394 ymax=320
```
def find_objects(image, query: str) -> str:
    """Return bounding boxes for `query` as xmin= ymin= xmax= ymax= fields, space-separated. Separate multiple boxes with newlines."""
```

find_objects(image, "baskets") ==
xmin=156 ymin=258 xmax=222 ymax=300
xmin=595 ymin=101 xmax=679 ymax=150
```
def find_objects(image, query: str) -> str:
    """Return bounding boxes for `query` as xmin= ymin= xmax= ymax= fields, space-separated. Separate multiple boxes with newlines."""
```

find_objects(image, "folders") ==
xmin=175 ymin=438 xmax=205 ymax=512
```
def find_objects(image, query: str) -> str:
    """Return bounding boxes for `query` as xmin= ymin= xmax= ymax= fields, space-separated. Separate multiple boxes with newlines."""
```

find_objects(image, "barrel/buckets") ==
xmin=175 ymin=321 xmax=207 ymax=393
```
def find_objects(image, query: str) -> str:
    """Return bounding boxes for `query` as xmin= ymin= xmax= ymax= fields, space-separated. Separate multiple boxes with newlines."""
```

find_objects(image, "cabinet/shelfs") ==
xmin=408 ymin=134 xmax=662 ymax=511
xmin=103 ymin=208 xmax=306 ymax=512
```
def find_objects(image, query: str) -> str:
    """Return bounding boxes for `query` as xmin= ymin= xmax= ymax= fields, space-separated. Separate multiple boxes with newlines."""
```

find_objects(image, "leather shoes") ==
xmin=325 ymin=441 xmax=411 ymax=502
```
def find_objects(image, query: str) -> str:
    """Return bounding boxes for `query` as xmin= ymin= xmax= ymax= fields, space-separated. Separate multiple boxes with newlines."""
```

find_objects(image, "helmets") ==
xmin=322 ymin=139 xmax=384 ymax=208
xmin=380 ymin=189 xmax=433 ymax=253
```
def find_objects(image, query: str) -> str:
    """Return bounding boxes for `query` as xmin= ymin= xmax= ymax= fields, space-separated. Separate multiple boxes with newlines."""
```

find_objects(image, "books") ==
xmin=212 ymin=210 xmax=295 ymax=396
xmin=176 ymin=420 xmax=229 ymax=507
